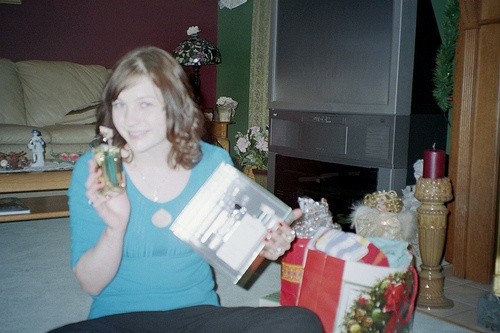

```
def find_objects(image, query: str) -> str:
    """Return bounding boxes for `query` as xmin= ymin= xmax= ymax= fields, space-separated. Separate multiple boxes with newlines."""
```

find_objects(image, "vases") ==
xmin=219 ymin=106 xmax=231 ymax=123
xmin=243 ymin=165 xmax=257 ymax=181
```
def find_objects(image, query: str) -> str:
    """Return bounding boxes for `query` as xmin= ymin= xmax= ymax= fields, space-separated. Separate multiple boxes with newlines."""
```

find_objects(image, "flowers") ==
xmin=215 ymin=95 xmax=239 ymax=118
xmin=232 ymin=125 xmax=269 ymax=171
xmin=50 ymin=151 xmax=83 ymax=164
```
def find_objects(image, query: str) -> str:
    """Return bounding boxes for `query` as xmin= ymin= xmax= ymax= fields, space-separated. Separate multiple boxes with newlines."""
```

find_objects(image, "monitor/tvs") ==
xmin=268 ymin=0 xmax=418 ymax=115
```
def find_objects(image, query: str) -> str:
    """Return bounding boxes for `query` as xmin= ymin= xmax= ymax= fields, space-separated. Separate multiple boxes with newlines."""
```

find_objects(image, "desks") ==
xmin=213 ymin=119 xmax=236 ymax=154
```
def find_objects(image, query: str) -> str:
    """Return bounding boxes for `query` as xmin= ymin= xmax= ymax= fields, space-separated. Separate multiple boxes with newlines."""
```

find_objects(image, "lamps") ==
xmin=170 ymin=25 xmax=223 ymax=113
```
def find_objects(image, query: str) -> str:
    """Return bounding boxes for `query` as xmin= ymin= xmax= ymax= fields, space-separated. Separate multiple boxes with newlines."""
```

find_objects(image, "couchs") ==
xmin=0 ymin=58 xmax=115 ymax=158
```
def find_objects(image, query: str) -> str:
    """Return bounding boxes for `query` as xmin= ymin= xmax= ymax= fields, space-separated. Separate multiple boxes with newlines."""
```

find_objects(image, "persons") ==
xmin=27 ymin=130 xmax=45 ymax=163
xmin=67 ymin=47 xmax=304 ymax=322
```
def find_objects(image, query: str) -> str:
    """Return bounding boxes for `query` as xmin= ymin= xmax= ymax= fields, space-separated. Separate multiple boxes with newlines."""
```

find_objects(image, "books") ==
xmin=169 ymin=161 xmax=297 ymax=291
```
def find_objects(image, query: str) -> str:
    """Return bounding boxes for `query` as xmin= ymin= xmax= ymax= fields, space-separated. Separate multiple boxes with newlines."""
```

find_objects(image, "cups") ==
xmin=95 ymin=142 xmax=127 ymax=199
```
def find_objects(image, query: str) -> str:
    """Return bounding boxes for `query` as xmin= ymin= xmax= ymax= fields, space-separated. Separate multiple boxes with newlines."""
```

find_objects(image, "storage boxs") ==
xmin=169 ymin=160 xmax=297 ymax=292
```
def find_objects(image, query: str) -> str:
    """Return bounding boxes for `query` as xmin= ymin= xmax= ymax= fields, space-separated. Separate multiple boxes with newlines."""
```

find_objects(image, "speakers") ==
xmin=267 ymin=110 xmax=411 ymax=169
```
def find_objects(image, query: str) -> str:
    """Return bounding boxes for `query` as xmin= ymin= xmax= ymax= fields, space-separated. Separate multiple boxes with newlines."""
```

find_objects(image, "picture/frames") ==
xmin=0 ymin=0 xmax=22 ymax=5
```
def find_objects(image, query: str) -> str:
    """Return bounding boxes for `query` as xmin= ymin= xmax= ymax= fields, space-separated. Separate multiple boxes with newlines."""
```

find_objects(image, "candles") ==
xmin=423 ymin=142 xmax=447 ymax=178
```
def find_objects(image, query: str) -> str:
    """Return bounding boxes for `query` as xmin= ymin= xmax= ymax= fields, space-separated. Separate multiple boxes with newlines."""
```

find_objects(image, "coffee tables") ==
xmin=0 ymin=159 xmax=72 ymax=223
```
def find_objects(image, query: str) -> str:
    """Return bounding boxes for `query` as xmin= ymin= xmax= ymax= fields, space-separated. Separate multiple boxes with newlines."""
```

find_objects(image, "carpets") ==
xmin=0 ymin=216 xmax=477 ymax=333
